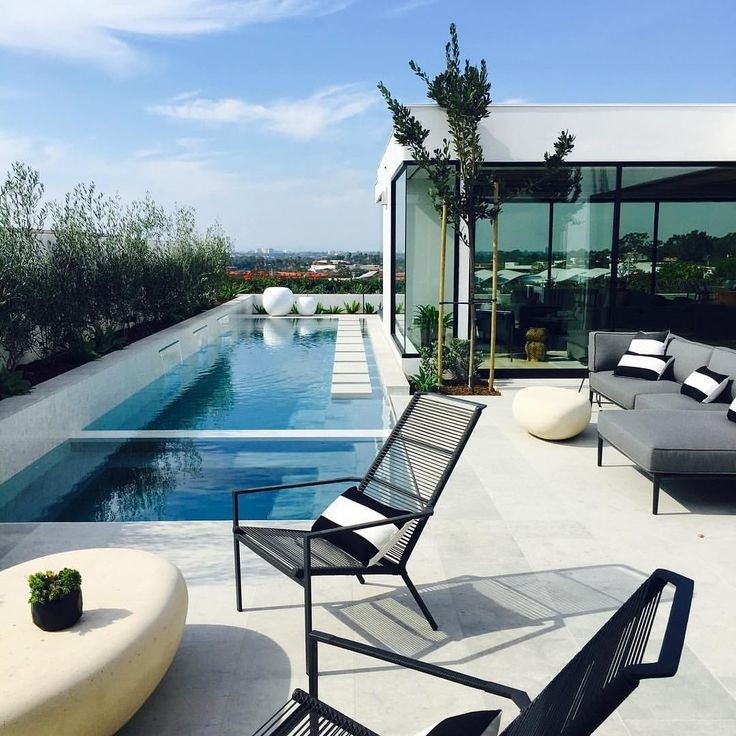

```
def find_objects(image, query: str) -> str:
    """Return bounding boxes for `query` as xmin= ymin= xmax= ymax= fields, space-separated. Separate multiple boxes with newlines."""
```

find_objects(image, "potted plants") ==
xmin=23 ymin=565 xmax=86 ymax=633
xmin=412 ymin=304 xmax=457 ymax=348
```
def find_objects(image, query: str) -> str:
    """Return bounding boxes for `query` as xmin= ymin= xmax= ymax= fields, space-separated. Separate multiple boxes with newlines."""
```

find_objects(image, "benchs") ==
xmin=586 ymin=326 xmax=736 ymax=517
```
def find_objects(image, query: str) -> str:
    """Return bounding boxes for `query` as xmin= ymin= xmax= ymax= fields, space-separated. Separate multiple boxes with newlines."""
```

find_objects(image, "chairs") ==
xmin=225 ymin=387 xmax=493 ymax=680
xmin=249 ymin=566 xmax=698 ymax=736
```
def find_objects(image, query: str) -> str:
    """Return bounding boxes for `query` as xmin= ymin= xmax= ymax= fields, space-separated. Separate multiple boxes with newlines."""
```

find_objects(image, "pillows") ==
xmin=627 ymin=329 xmax=670 ymax=356
xmin=725 ymin=397 xmax=736 ymax=424
xmin=680 ymin=363 xmax=730 ymax=404
xmin=610 ymin=351 xmax=675 ymax=381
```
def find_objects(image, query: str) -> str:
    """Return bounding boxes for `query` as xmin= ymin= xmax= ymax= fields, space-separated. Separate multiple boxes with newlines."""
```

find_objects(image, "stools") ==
xmin=508 ymin=384 xmax=592 ymax=443
xmin=0 ymin=544 xmax=191 ymax=736
